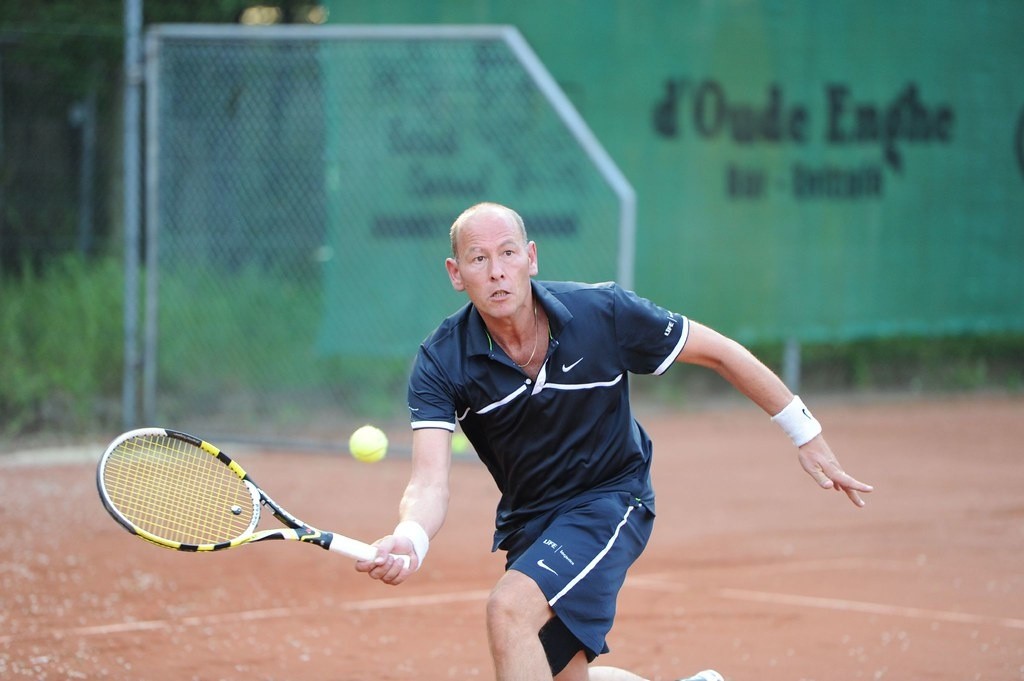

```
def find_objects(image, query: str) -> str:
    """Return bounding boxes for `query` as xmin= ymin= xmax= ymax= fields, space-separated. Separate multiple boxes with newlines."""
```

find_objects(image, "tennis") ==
xmin=346 ymin=423 xmax=390 ymax=465
xmin=449 ymin=431 xmax=471 ymax=456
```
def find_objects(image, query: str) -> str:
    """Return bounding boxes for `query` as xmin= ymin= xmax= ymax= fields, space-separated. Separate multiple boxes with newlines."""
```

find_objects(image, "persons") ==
xmin=355 ymin=202 xmax=873 ymax=681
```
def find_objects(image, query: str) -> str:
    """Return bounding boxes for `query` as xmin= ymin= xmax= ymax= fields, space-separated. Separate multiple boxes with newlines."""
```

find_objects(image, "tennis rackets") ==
xmin=93 ymin=423 xmax=412 ymax=576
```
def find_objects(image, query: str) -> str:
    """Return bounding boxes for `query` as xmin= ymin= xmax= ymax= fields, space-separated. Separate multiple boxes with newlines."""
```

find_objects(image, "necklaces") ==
xmin=516 ymin=294 xmax=538 ymax=368
